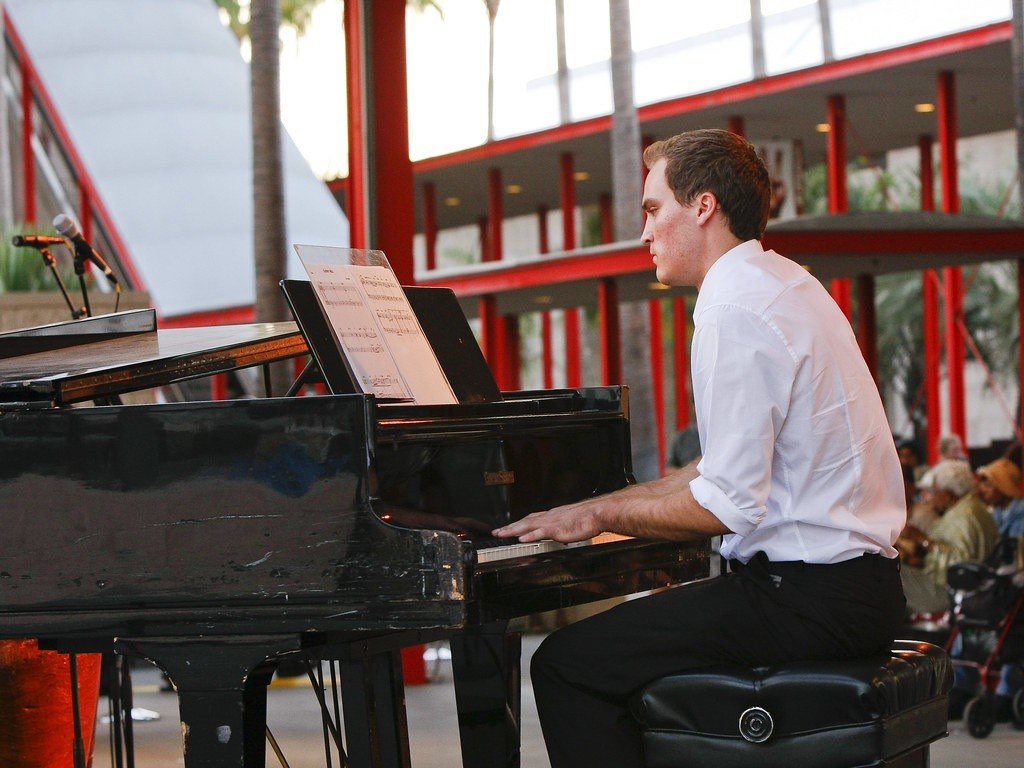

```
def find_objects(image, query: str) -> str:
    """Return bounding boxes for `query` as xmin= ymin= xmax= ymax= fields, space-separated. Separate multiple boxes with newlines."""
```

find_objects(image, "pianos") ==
xmin=0 ymin=290 xmax=711 ymax=768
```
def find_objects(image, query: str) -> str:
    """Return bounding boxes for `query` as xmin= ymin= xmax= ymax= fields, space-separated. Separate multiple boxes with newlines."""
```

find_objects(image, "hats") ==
xmin=976 ymin=456 xmax=1019 ymax=498
xmin=916 ymin=459 xmax=974 ymax=496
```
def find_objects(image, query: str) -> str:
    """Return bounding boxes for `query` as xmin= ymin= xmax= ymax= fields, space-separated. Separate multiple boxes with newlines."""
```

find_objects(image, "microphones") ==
xmin=52 ymin=214 xmax=118 ymax=284
xmin=12 ymin=235 xmax=66 ymax=248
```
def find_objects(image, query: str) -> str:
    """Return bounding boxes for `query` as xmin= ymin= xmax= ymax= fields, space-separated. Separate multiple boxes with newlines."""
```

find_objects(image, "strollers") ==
xmin=942 ymin=512 xmax=1024 ymax=740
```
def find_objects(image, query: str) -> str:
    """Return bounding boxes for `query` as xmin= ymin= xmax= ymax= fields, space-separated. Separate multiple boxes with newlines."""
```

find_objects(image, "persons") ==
xmin=489 ymin=125 xmax=907 ymax=767
xmin=898 ymin=434 xmax=1024 ymax=614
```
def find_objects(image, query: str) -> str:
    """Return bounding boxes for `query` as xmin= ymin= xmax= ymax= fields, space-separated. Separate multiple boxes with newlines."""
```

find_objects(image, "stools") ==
xmin=634 ymin=638 xmax=954 ymax=768
